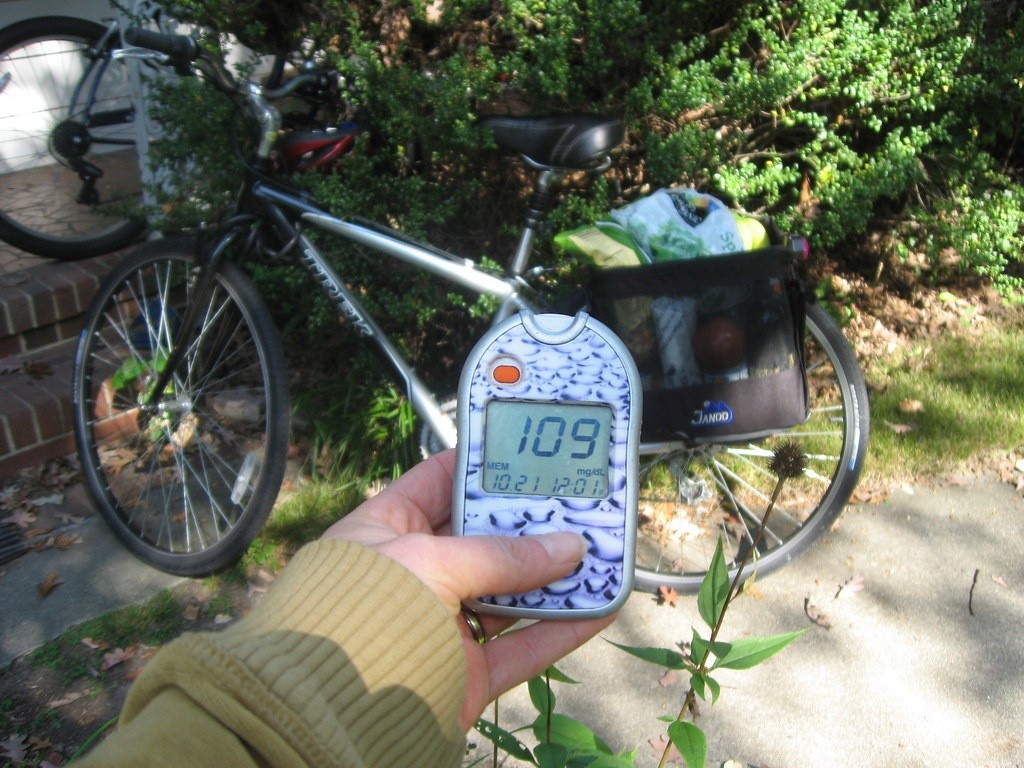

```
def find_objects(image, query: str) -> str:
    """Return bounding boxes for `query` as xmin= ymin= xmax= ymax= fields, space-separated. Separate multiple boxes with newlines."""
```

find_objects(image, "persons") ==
xmin=69 ymin=447 xmax=621 ymax=768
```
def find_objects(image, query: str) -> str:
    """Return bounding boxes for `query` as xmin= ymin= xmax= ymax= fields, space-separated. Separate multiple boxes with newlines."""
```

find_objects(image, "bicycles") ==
xmin=69 ymin=29 xmax=874 ymax=594
xmin=0 ymin=9 xmax=437 ymax=263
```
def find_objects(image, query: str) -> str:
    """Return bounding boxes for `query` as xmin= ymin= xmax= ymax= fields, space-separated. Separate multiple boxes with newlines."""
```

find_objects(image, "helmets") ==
xmin=276 ymin=122 xmax=376 ymax=176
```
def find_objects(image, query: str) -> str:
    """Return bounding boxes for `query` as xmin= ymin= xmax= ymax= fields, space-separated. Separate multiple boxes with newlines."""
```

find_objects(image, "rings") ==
xmin=462 ymin=608 xmax=486 ymax=644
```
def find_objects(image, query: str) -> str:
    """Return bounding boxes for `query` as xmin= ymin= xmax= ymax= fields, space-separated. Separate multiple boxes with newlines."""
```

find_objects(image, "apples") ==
xmin=691 ymin=315 xmax=748 ymax=372
